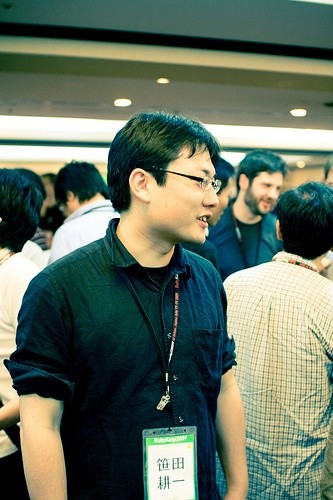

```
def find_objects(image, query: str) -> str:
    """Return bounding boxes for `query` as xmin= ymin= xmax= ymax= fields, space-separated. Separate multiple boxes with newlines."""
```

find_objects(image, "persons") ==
xmin=0 ymin=113 xmax=331 ymax=494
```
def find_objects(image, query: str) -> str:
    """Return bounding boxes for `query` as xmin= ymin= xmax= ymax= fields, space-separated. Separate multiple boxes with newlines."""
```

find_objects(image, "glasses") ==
xmin=151 ymin=166 xmax=221 ymax=195
